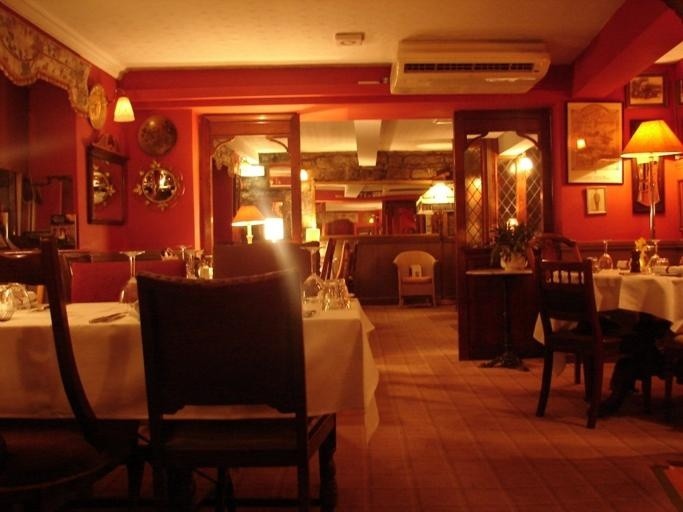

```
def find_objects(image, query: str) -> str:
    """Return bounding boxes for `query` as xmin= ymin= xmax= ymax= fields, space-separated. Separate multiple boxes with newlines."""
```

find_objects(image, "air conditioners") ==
xmin=390 ymin=40 xmax=551 ymax=94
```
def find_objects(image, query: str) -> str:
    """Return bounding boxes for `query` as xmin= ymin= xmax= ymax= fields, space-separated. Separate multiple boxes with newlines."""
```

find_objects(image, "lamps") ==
xmin=232 ymin=205 xmax=265 ymax=244
xmin=87 ymin=83 xmax=135 ymax=129
xmin=422 ymin=181 xmax=455 ymax=204
xmin=621 ymin=120 xmax=683 ymax=242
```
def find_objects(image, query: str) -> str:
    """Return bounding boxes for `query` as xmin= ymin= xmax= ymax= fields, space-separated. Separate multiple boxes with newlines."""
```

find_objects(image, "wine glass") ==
xmin=119 ymin=251 xmax=146 ymax=317
xmin=648 ymin=239 xmax=661 ymax=274
xmin=300 ymin=246 xmax=325 ymax=305
xmin=597 ymin=239 xmax=613 ymax=272
xmin=184 ymin=249 xmax=204 ymax=279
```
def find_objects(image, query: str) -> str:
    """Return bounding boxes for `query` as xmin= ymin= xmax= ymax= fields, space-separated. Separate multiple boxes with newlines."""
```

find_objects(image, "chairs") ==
xmin=394 ymin=251 xmax=438 ymax=307
xmin=528 ymin=233 xmax=683 ymax=428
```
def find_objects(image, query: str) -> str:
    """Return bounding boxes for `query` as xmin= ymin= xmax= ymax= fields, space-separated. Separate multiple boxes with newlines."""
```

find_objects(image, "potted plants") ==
xmin=488 ymin=212 xmax=540 ymax=271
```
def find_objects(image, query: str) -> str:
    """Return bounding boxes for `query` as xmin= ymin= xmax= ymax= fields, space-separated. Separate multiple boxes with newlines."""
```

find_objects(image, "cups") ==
xmin=657 ymin=258 xmax=669 ymax=267
xmin=325 ymin=277 xmax=351 ymax=311
xmin=616 ymin=260 xmax=629 ymax=272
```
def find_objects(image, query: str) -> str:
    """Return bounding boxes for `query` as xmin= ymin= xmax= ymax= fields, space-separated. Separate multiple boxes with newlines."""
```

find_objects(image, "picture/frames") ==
xmin=585 ymin=186 xmax=608 ymax=215
xmin=629 ymin=120 xmax=665 ymax=214
xmin=566 ymin=101 xmax=624 ymax=185
xmin=625 ymin=72 xmax=670 ymax=108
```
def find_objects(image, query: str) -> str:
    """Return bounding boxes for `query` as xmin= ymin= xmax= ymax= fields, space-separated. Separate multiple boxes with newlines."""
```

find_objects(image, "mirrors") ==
xmin=87 ymin=133 xmax=130 ymax=222
xmin=133 ymin=160 xmax=184 ymax=212
